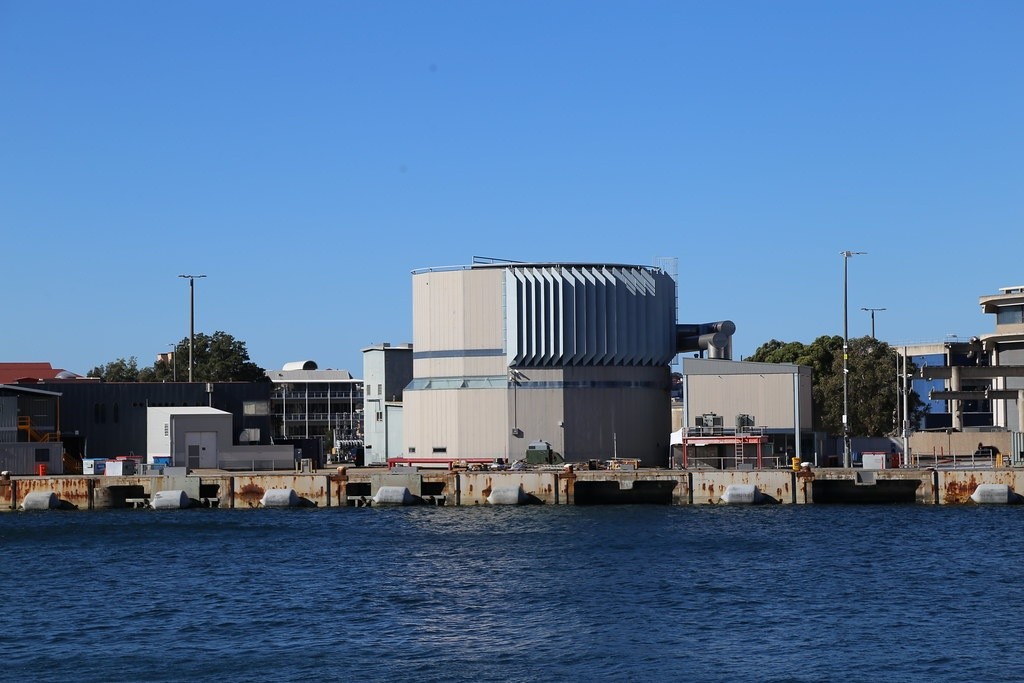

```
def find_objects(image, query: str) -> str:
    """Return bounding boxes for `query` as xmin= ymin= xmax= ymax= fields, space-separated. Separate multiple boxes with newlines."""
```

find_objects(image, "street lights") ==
xmin=860 ymin=308 xmax=886 ymax=338
xmin=177 ymin=274 xmax=207 ymax=382
xmin=841 ymin=250 xmax=868 ymax=468
xmin=166 ymin=343 xmax=176 ymax=382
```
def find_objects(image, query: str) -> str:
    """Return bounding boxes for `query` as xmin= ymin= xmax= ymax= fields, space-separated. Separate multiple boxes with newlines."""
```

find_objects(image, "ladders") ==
xmin=734 ymin=427 xmax=743 ymax=470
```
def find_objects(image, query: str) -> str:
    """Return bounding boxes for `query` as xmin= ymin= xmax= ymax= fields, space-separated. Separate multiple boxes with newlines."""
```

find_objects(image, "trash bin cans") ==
xmin=152 ymin=456 xmax=172 ymax=471
xmin=792 ymin=458 xmax=800 ymax=472
xmin=40 ymin=464 xmax=47 ymax=476
